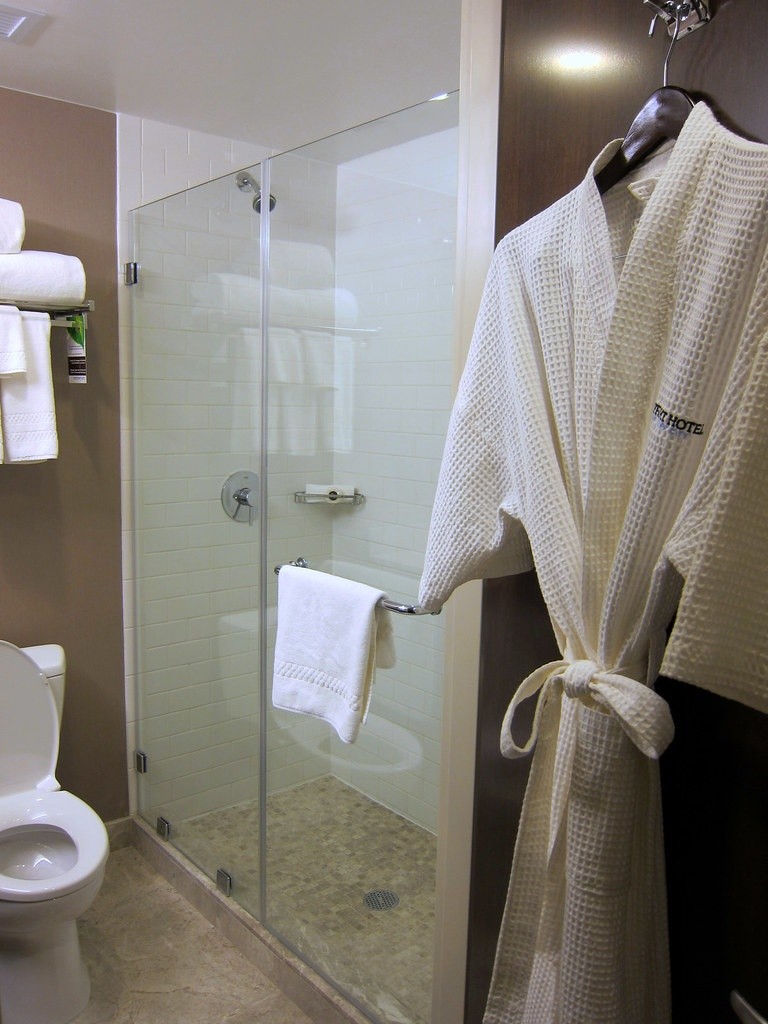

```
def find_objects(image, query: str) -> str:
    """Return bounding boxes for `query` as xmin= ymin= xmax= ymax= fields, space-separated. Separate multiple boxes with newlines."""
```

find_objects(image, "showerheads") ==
xmin=236 ymin=170 xmax=277 ymax=216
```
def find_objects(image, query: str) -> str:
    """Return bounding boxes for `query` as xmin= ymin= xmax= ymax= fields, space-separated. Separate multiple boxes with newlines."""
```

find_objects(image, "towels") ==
xmin=271 ymin=564 xmax=399 ymax=744
xmin=0 ymin=196 xmax=87 ymax=466
xmin=202 ymin=238 xmax=361 ymax=456
xmin=303 ymin=482 xmax=357 ymax=505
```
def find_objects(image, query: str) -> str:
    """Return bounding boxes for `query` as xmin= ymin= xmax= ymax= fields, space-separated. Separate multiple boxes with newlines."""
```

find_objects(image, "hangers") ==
xmin=484 ymin=1 xmax=768 ymax=285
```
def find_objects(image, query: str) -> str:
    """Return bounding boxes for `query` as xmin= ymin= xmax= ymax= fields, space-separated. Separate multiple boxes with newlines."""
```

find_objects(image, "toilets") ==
xmin=0 ymin=638 xmax=112 ymax=1024
xmin=217 ymin=601 xmax=425 ymax=777
